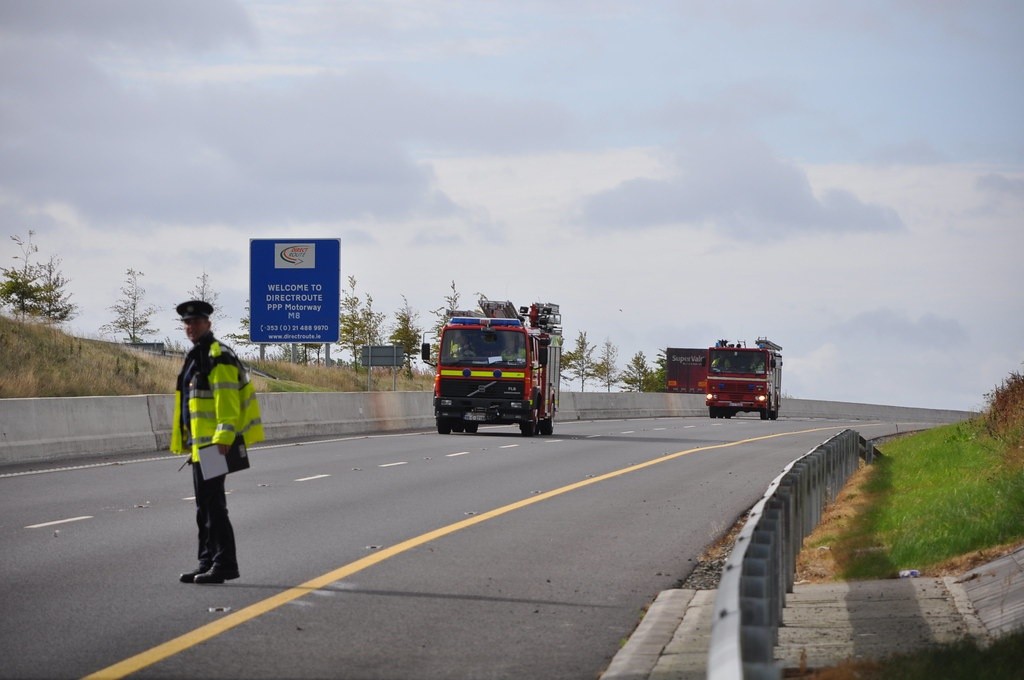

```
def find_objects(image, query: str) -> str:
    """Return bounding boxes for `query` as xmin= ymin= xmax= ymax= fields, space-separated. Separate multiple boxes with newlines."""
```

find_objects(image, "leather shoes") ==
xmin=195 ymin=561 xmax=238 ymax=585
xmin=182 ymin=561 xmax=213 ymax=584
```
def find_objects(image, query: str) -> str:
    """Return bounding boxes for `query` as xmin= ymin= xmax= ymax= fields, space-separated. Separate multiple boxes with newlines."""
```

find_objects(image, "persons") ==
xmin=450 ymin=335 xmax=475 ymax=356
xmin=735 ymin=354 xmax=765 ymax=372
xmin=170 ymin=301 xmax=265 ymax=583
xmin=450 ymin=330 xmax=472 ymax=351
xmin=711 ymin=353 xmax=731 ymax=369
xmin=502 ymin=338 xmax=526 ymax=360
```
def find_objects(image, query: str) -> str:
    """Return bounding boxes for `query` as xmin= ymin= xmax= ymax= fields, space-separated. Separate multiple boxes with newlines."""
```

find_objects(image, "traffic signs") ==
xmin=248 ymin=235 xmax=342 ymax=344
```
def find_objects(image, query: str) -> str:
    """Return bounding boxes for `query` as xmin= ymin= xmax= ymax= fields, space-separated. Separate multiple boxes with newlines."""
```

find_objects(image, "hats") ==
xmin=178 ymin=301 xmax=214 ymax=322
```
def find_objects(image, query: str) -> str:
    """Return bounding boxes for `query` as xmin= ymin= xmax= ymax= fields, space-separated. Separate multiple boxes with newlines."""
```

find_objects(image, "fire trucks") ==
xmin=419 ymin=298 xmax=563 ymax=436
xmin=704 ymin=339 xmax=785 ymax=420
